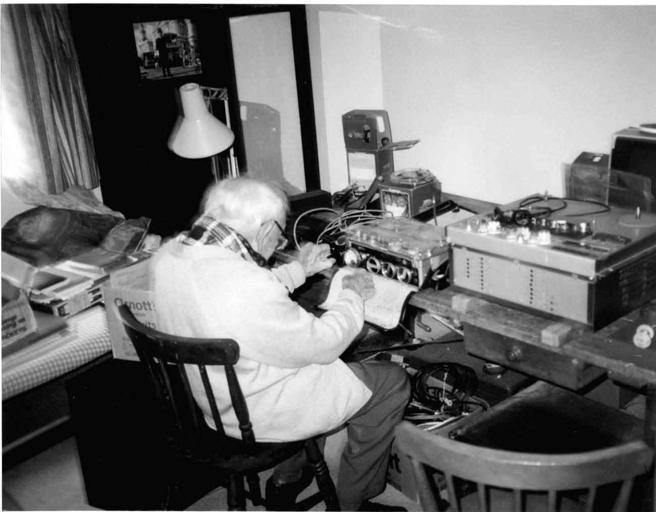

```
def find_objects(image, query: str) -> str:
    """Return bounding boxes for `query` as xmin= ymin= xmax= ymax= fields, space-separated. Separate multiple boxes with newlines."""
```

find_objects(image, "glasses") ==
xmin=261 ymin=220 xmax=291 ymax=251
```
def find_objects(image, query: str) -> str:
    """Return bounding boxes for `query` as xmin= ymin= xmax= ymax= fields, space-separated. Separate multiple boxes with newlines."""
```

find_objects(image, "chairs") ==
xmin=112 ymin=304 xmax=339 ymax=509
xmin=397 ymin=420 xmax=654 ymax=511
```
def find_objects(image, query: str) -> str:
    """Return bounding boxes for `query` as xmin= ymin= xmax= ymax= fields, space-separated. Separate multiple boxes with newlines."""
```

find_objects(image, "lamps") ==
xmin=168 ymin=84 xmax=237 ymax=179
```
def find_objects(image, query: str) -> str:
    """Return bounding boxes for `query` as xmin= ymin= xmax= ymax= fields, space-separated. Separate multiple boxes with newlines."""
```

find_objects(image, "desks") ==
xmin=297 ymin=268 xmax=655 ymax=441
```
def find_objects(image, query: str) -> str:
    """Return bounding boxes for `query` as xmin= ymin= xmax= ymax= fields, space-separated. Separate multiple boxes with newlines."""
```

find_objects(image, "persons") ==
xmin=143 ymin=171 xmax=406 ymax=512
xmin=137 ymin=30 xmax=154 ymax=69
xmin=155 ymin=26 xmax=174 ymax=77
